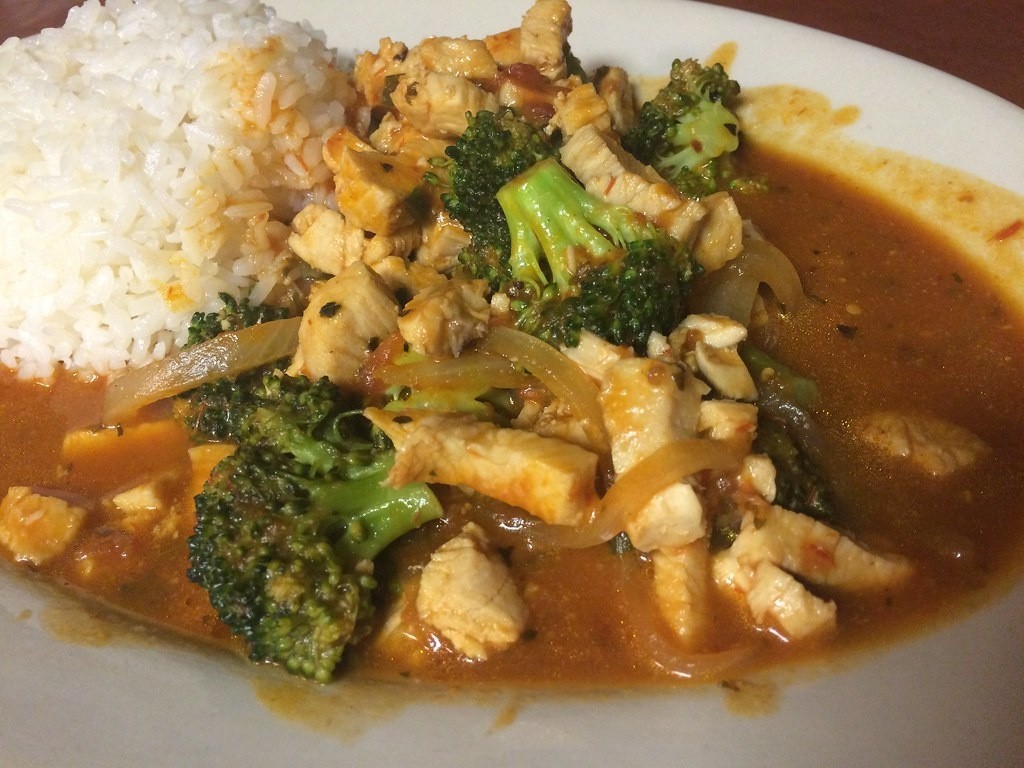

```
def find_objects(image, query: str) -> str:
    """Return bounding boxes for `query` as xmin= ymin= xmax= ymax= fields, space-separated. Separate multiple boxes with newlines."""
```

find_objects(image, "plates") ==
xmin=1 ymin=0 xmax=1024 ymax=767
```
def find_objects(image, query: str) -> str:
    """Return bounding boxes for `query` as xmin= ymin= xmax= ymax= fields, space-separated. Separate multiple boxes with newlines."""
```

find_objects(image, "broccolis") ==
xmin=165 ymin=61 xmax=834 ymax=683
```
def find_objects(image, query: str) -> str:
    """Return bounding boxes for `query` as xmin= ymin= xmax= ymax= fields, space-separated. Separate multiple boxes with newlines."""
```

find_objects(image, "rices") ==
xmin=0 ymin=0 xmax=380 ymax=384
